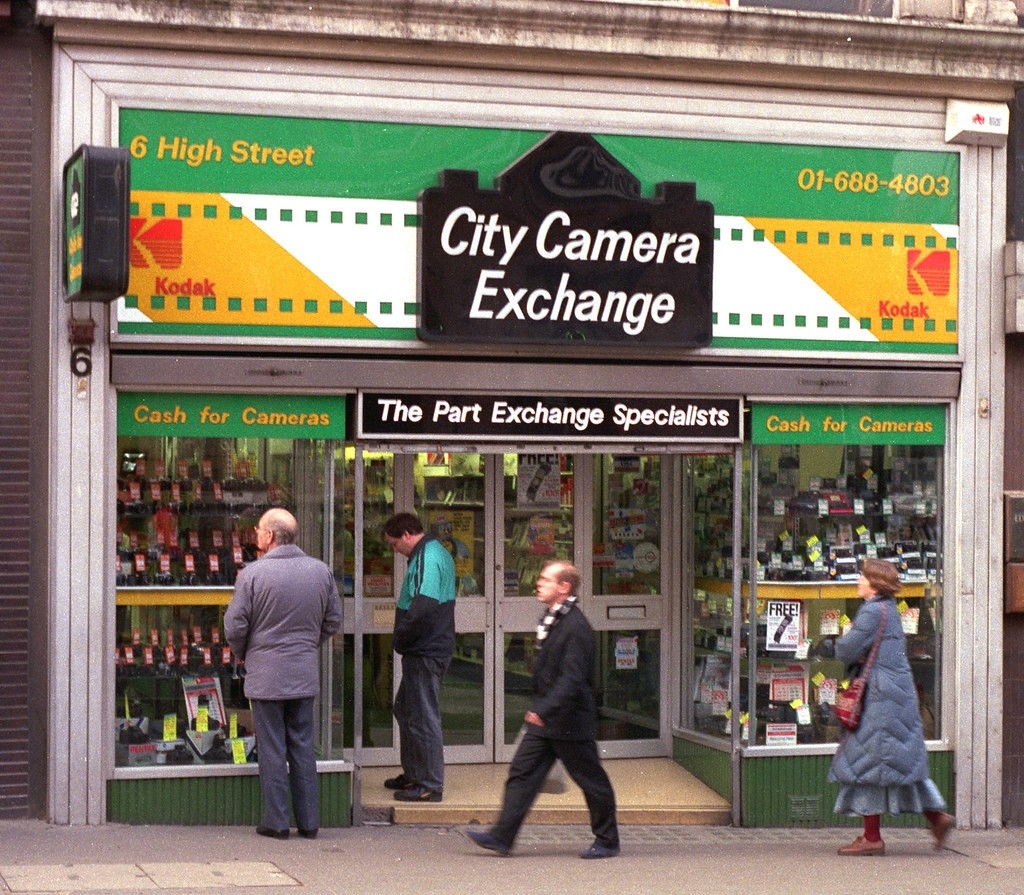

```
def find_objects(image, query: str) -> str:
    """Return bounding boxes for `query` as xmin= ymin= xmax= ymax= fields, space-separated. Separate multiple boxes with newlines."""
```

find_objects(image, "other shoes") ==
xmin=838 ymin=836 xmax=885 ymax=855
xmin=932 ymin=812 xmax=955 ymax=850
xmin=298 ymin=828 xmax=319 ymax=839
xmin=257 ymin=826 xmax=289 ymax=838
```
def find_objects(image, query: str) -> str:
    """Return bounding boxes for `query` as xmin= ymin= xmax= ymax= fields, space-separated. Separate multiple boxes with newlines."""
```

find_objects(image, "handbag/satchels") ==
xmin=835 ymin=676 xmax=866 ymax=731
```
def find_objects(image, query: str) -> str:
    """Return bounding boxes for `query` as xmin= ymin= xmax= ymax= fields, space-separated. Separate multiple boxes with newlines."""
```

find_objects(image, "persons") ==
xmin=832 ymin=556 xmax=957 ymax=858
xmin=461 ymin=560 xmax=622 ymax=860
xmin=380 ymin=508 xmax=456 ymax=805
xmin=220 ymin=506 xmax=345 ymax=840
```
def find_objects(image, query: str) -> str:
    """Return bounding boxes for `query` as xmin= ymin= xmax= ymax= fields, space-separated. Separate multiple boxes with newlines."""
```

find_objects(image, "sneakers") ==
xmin=394 ymin=784 xmax=443 ymax=802
xmin=384 ymin=774 xmax=414 ymax=789
xmin=580 ymin=842 xmax=622 ymax=858
xmin=468 ymin=830 xmax=509 ymax=855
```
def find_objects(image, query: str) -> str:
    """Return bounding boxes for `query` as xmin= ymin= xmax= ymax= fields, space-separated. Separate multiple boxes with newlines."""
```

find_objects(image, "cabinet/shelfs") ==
xmin=354 ymin=454 xmax=579 ymax=684
